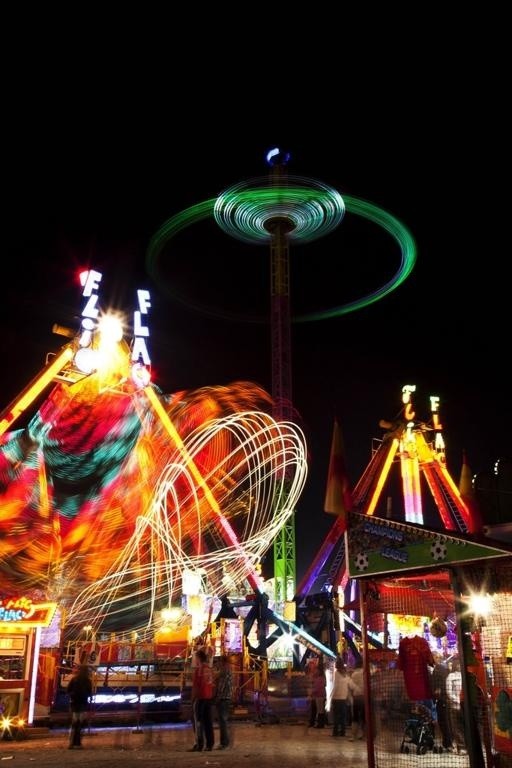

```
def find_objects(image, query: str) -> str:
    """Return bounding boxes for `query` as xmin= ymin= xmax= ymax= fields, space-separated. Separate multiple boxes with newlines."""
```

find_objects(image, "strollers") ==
xmin=400 ymin=704 xmax=443 ymax=755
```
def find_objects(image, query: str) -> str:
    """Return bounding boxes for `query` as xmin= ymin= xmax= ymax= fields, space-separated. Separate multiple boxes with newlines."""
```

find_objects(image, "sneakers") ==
xmin=185 ymin=743 xmax=230 ymax=752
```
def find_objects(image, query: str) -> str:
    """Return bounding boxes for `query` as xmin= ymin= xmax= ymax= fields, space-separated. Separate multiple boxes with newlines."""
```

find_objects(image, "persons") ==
xmin=347 ymin=661 xmax=366 ymax=742
xmin=330 ymin=658 xmax=362 ymax=737
xmin=430 ymin=651 xmax=456 ymax=753
xmin=303 ymin=661 xmax=320 ymax=728
xmin=310 ymin=669 xmax=327 ymax=728
xmin=213 ymin=656 xmax=234 ymax=751
xmin=445 ymin=657 xmax=467 ymax=755
xmin=66 ymin=664 xmax=93 ymax=750
xmin=185 ymin=650 xmax=216 ymax=753
